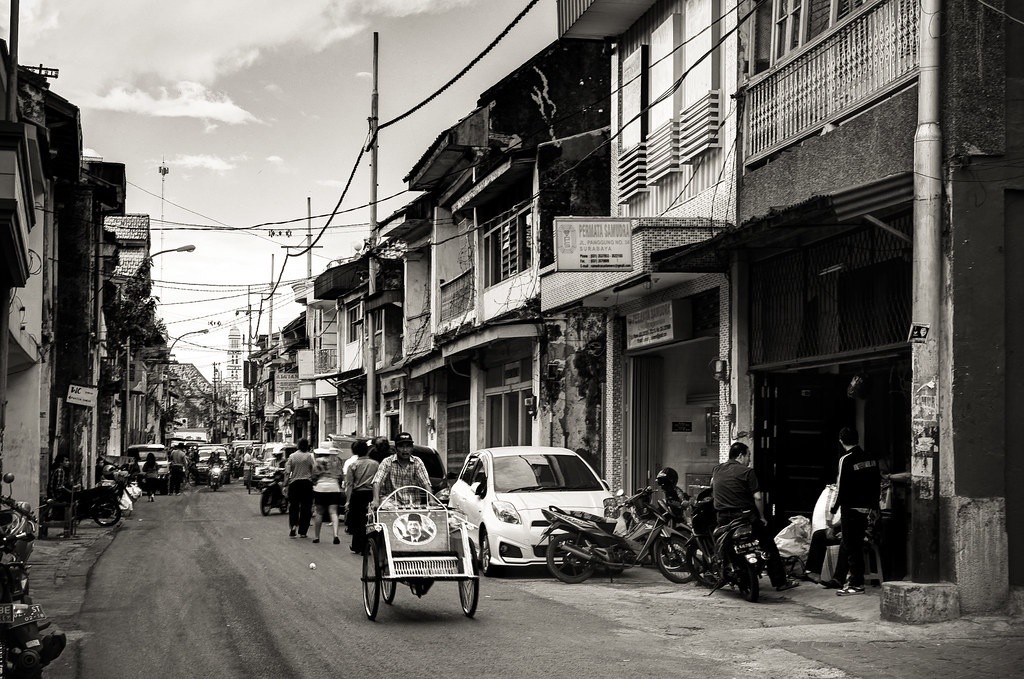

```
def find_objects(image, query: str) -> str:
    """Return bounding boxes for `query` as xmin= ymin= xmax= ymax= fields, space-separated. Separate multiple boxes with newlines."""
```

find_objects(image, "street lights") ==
xmin=125 ymin=244 xmax=197 ymax=453
xmin=164 ymin=329 xmax=210 ymax=447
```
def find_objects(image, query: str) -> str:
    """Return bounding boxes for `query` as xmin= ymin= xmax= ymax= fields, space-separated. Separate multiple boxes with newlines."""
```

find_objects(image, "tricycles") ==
xmin=355 ymin=485 xmax=480 ymax=621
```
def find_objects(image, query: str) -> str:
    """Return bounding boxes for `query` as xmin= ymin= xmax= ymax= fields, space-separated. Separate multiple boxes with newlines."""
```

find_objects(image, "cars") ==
xmin=445 ymin=444 xmax=629 ymax=577
xmin=123 ymin=426 xmax=451 ymax=529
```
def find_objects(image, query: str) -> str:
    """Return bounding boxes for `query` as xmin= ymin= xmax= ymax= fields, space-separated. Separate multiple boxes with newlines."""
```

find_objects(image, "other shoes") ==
xmin=350 ymin=545 xmax=359 ymax=554
xmin=333 ymin=536 xmax=340 ymax=543
xmin=836 ymin=586 xmax=865 ymax=596
xmin=817 ymin=579 xmax=841 ymax=589
xmin=289 ymin=530 xmax=296 ymax=536
xmin=298 ymin=533 xmax=305 ymax=537
xmin=313 ymin=538 xmax=320 ymax=542
xmin=151 ymin=495 xmax=154 ymax=502
xmin=773 ymin=580 xmax=799 ymax=591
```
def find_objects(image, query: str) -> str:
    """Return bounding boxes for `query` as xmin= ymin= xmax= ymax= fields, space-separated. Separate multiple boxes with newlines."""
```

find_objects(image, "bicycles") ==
xmin=259 ymin=467 xmax=288 ymax=516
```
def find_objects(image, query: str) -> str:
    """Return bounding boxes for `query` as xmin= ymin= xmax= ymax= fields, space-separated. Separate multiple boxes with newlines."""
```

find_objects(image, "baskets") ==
xmin=257 ymin=480 xmax=275 ymax=490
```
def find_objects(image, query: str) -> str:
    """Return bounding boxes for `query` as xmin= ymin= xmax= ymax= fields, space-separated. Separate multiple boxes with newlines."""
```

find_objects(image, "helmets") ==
xmin=656 ymin=468 xmax=679 ymax=493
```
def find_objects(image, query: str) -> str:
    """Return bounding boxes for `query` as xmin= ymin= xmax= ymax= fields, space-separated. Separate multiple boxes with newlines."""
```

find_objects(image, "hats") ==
xmin=313 ymin=440 xmax=339 ymax=454
xmin=395 ymin=432 xmax=414 ymax=446
xmin=272 ymin=451 xmax=284 ymax=455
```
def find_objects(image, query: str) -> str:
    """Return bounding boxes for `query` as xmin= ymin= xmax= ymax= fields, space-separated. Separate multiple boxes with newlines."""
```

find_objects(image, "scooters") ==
xmin=207 ymin=463 xmax=225 ymax=492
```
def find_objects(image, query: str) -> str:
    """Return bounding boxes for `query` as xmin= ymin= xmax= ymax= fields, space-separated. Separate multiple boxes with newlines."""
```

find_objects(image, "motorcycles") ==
xmin=61 ymin=476 xmax=122 ymax=527
xmin=0 ymin=471 xmax=68 ymax=679
xmin=533 ymin=469 xmax=772 ymax=602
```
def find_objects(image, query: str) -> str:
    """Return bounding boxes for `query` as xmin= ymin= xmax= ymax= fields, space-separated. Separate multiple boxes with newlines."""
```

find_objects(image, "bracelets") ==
xmin=888 ymin=475 xmax=890 ymax=480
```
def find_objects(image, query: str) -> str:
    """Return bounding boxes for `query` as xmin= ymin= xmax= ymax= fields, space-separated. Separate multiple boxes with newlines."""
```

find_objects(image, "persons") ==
xmin=282 ymin=439 xmax=315 ymax=538
xmin=95 ymin=458 xmax=104 ymax=484
xmin=798 ymin=506 xmax=881 ymax=590
xmin=312 ymin=441 xmax=343 ymax=544
xmin=710 ymin=442 xmax=800 ymax=591
xmin=142 ymin=453 xmax=158 ymax=502
xmin=342 ymin=436 xmax=390 ymax=555
xmin=269 ymin=448 xmax=285 ymax=468
xmin=885 ymin=473 xmax=906 ymax=481
xmin=816 ymin=428 xmax=881 ymax=595
xmin=47 ymin=455 xmax=70 ymax=499
xmin=167 ymin=443 xmax=253 ymax=496
xmin=371 ymin=432 xmax=438 ymax=508
